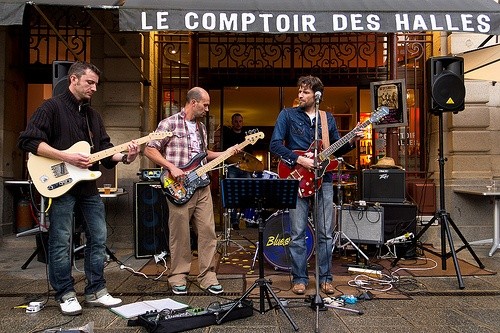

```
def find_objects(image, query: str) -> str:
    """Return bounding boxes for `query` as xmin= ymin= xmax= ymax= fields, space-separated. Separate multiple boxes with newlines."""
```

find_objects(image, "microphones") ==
xmin=315 ymin=91 xmax=321 ymax=99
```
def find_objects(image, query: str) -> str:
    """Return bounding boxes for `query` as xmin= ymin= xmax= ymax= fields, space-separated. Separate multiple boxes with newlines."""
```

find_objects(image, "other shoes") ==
xmin=246 ymin=222 xmax=259 ymax=228
xmin=233 ymin=223 xmax=240 ymax=230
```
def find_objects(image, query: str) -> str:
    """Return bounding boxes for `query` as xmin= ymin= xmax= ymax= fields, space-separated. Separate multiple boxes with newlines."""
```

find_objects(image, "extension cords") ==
xmin=348 ymin=267 xmax=382 ymax=279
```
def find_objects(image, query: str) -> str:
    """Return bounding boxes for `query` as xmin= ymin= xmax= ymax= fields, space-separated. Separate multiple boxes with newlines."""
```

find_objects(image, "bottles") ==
xmin=340 ymin=295 xmax=360 ymax=304
xmin=261 ymin=173 xmax=277 ymax=179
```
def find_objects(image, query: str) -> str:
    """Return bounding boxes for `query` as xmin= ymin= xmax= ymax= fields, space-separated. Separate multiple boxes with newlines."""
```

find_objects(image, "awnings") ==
xmin=1 ymin=0 xmax=500 ymax=37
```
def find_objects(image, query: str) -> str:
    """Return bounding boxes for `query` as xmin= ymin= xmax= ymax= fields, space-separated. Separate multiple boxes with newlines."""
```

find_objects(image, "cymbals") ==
xmin=226 ymin=150 xmax=264 ymax=173
xmin=326 ymin=165 xmax=356 ymax=172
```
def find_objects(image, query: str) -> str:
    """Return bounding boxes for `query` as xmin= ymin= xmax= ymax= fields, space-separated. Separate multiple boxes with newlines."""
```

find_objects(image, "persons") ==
xmin=143 ymin=87 xmax=242 ymax=295
xmin=269 ymin=74 xmax=367 ymax=294
xmin=17 ymin=59 xmax=142 ymax=316
xmin=222 ymin=113 xmax=262 ymax=230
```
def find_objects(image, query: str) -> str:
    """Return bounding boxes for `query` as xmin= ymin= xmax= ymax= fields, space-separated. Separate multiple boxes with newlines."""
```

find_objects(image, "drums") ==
xmin=259 ymin=209 xmax=317 ymax=272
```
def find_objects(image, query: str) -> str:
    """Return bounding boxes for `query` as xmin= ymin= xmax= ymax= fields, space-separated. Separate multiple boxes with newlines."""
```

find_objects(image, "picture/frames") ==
xmin=370 ymin=78 xmax=408 ymax=129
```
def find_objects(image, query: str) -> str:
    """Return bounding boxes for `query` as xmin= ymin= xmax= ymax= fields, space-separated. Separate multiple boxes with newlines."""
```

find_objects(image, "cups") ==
xmin=104 ymin=184 xmax=111 ymax=194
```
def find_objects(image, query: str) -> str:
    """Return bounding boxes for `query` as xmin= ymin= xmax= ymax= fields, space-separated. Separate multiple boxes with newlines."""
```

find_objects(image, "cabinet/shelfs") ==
xmin=332 ymin=113 xmax=358 ymax=200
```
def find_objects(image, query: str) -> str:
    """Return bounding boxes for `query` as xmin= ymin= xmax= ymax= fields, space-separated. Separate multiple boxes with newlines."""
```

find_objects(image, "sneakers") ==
xmin=171 ymin=285 xmax=187 ymax=294
xmin=208 ymin=283 xmax=224 ymax=294
xmin=85 ymin=293 xmax=122 ymax=308
xmin=60 ymin=297 xmax=82 ymax=316
xmin=320 ymin=282 xmax=334 ymax=294
xmin=292 ymin=283 xmax=306 ymax=294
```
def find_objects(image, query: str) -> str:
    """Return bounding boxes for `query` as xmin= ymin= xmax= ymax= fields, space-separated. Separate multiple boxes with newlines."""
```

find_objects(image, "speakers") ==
xmin=338 ymin=169 xmax=417 ymax=258
xmin=53 ymin=61 xmax=75 ymax=96
xmin=133 ymin=181 xmax=198 ymax=259
xmin=426 ymin=56 xmax=465 ymax=116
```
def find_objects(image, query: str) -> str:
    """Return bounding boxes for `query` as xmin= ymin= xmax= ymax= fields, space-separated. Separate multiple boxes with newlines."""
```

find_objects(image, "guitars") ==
xmin=25 ymin=130 xmax=174 ymax=199
xmin=277 ymin=106 xmax=390 ymax=199
xmin=159 ymin=128 xmax=265 ymax=206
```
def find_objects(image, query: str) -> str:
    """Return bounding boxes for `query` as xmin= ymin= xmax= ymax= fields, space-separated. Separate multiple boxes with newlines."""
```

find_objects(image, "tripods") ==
xmin=304 ymin=100 xmax=364 ymax=333
xmin=391 ymin=113 xmax=485 ymax=290
xmin=209 ymin=158 xmax=300 ymax=332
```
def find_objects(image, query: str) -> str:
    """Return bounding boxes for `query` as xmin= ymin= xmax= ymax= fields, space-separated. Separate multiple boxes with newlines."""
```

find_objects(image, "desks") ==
xmin=73 ymin=191 xmax=128 ymax=262
xmin=4 ymin=180 xmax=49 ymax=237
xmin=454 ymin=189 xmax=500 ymax=256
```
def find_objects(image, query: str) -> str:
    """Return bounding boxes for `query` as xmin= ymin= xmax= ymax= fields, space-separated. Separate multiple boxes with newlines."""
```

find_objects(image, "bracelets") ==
xmin=121 ymin=153 xmax=130 ymax=165
